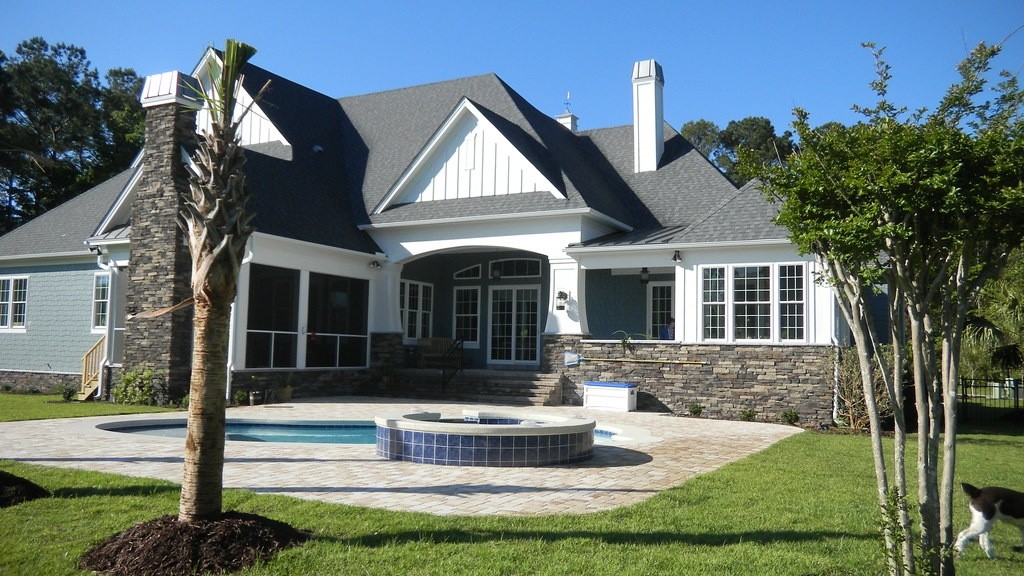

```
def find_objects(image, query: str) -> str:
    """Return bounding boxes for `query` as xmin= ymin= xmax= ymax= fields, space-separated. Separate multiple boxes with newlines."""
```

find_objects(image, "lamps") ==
xmin=672 ymin=250 xmax=682 ymax=261
xmin=556 ymin=290 xmax=567 ymax=310
xmin=87 ymin=245 xmax=103 ymax=256
xmin=369 ymin=261 xmax=382 ymax=274
xmin=494 ymin=249 xmax=500 ymax=280
xmin=640 ymin=268 xmax=650 ymax=284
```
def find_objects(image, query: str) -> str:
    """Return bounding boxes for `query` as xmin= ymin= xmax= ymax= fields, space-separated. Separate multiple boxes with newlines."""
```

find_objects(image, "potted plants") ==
xmin=234 ymin=375 xmax=294 ymax=406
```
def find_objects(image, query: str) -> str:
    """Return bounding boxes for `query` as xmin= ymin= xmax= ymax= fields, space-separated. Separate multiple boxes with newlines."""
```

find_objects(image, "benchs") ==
xmin=417 ymin=338 xmax=463 ymax=368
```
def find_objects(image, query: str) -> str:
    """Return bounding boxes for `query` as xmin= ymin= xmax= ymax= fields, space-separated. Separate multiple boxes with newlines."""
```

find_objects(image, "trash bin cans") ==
xmin=993 ymin=382 xmax=1002 ymax=400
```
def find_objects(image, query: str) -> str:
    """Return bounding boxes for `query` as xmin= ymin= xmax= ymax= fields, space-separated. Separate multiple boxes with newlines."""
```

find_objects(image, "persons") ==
xmin=659 ymin=318 xmax=674 ymax=340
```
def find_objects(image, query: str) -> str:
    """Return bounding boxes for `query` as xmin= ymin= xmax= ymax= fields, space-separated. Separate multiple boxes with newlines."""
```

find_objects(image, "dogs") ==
xmin=952 ymin=482 xmax=1024 ymax=559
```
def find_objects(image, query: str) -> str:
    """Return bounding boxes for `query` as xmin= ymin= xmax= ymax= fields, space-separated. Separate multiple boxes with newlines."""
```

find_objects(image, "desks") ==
xmin=403 ymin=349 xmax=417 ymax=369
